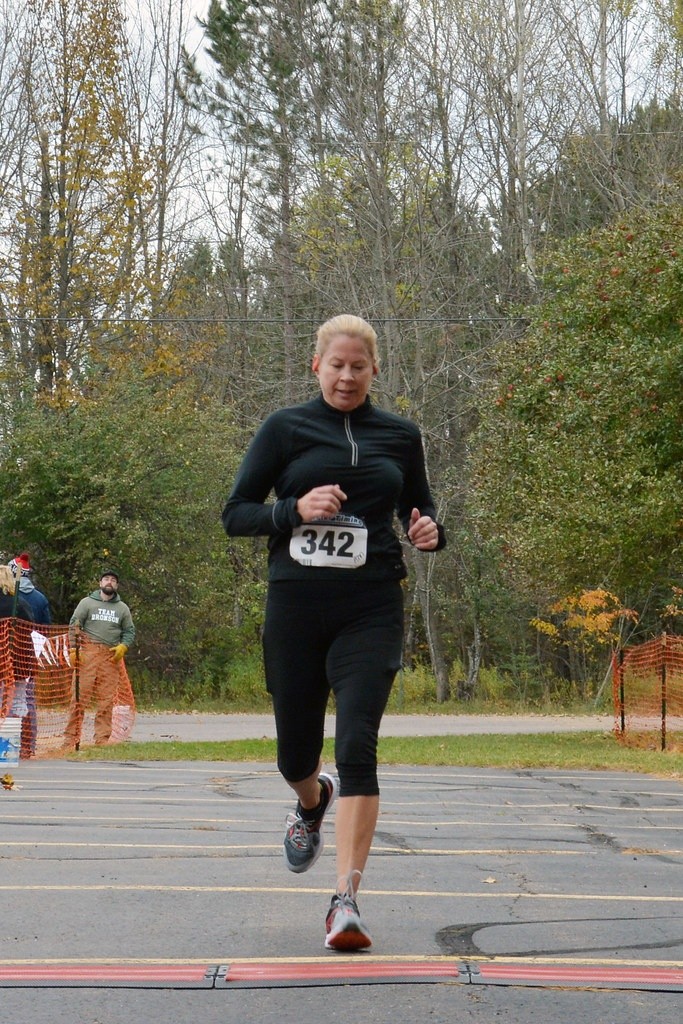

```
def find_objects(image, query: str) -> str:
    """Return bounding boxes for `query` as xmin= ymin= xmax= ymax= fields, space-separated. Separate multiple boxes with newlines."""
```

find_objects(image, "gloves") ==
xmin=108 ymin=644 xmax=128 ymax=664
xmin=69 ymin=647 xmax=83 ymax=668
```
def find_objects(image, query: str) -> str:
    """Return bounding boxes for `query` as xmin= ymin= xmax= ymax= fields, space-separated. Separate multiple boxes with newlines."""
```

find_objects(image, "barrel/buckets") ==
xmin=0 ymin=717 xmax=22 ymax=767
xmin=109 ymin=705 xmax=131 ymax=744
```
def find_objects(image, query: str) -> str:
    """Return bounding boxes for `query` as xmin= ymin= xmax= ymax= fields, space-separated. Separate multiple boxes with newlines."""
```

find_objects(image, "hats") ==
xmin=8 ymin=553 xmax=30 ymax=577
xmin=100 ymin=570 xmax=119 ymax=583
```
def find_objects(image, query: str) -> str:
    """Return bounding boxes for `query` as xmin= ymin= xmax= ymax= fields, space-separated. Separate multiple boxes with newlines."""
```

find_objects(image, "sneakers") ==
xmin=283 ymin=772 xmax=337 ymax=874
xmin=324 ymin=869 xmax=373 ymax=951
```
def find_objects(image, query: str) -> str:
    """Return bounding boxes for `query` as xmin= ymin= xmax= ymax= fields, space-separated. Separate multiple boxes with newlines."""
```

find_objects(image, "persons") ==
xmin=0 ymin=552 xmax=51 ymax=759
xmin=62 ymin=570 xmax=136 ymax=751
xmin=221 ymin=314 xmax=447 ymax=949
